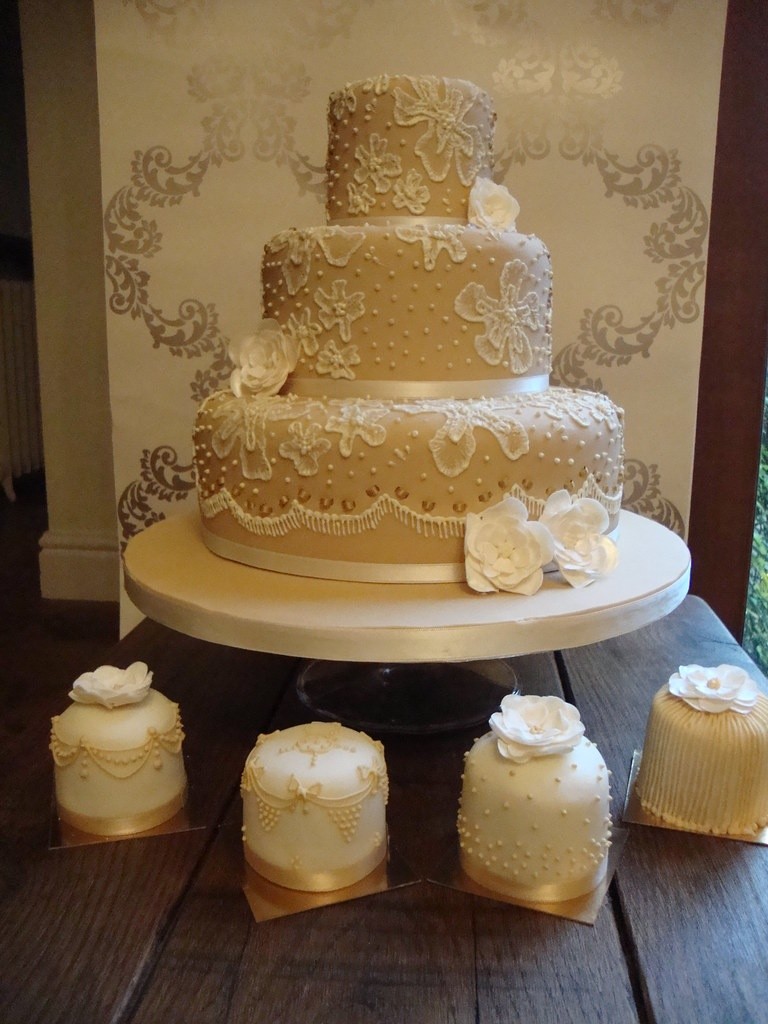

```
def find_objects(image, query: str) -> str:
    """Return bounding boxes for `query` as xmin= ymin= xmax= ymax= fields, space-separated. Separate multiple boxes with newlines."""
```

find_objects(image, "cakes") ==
xmin=242 ymin=720 xmax=387 ymax=887
xmin=55 ymin=665 xmax=186 ymax=838
xmin=649 ymin=662 xmax=768 ymax=838
xmin=199 ymin=75 xmax=628 ymax=599
xmin=461 ymin=692 xmax=613 ymax=902
